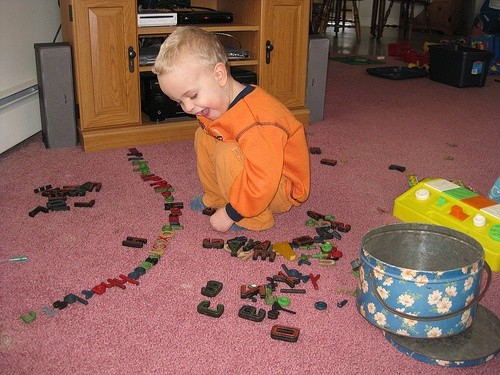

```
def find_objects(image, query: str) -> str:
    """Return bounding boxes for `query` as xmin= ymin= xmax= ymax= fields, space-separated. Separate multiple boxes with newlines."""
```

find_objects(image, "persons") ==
xmin=151 ymin=25 xmax=311 ymax=233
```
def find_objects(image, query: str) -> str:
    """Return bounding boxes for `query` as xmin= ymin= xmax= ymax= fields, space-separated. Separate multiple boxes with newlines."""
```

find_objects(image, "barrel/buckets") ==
xmin=357 ymin=222 xmax=492 ymax=338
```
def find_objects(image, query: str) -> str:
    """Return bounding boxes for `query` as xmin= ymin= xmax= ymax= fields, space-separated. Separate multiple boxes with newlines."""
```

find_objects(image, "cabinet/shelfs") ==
xmin=60 ymin=0 xmax=310 ymax=152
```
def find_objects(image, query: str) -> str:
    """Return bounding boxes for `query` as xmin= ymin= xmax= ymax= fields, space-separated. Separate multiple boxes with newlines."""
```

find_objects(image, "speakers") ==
xmin=34 ymin=42 xmax=77 ymax=149
xmin=304 ymin=33 xmax=330 ymax=123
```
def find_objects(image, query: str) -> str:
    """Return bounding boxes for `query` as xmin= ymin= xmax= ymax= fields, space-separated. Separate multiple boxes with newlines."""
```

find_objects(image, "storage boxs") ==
xmin=427 ymin=44 xmax=496 ymax=89
